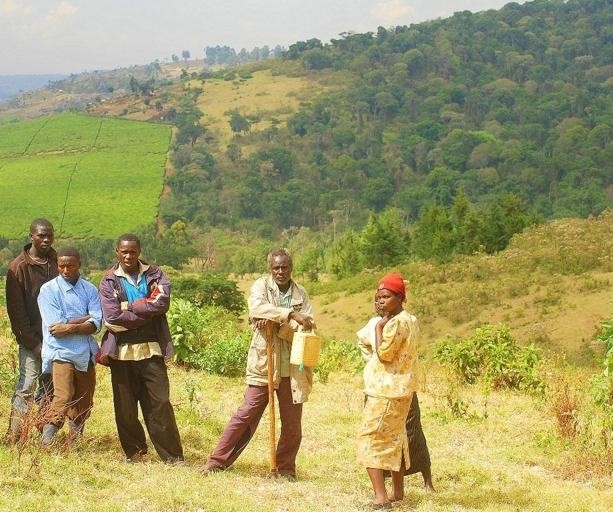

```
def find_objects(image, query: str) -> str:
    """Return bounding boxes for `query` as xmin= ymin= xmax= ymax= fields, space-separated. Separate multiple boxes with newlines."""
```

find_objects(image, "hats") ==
xmin=378 ymin=272 xmax=406 ymax=302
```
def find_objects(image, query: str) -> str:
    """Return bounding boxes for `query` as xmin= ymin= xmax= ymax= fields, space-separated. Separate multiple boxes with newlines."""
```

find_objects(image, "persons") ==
xmin=355 ymin=290 xmax=437 ymax=496
xmin=198 ymin=248 xmax=320 ymax=482
xmin=34 ymin=245 xmax=102 ymax=453
xmin=96 ymin=232 xmax=190 ymax=467
xmin=358 ymin=270 xmax=421 ymax=511
xmin=0 ymin=218 xmax=61 ymax=447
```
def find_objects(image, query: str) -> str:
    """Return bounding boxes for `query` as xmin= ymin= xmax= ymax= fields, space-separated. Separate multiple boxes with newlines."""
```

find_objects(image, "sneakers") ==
xmin=360 ymin=499 xmax=403 ymax=512
